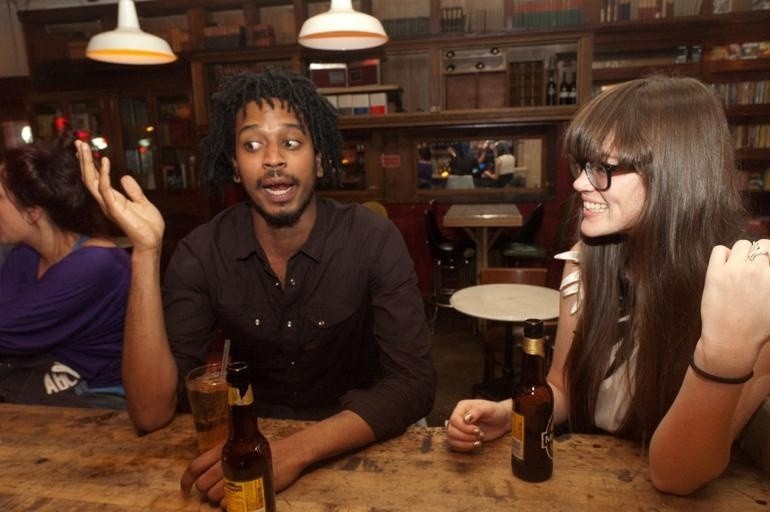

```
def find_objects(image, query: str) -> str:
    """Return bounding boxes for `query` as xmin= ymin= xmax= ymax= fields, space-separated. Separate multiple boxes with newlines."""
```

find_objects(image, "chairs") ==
xmin=423 ymin=259 xmax=476 ymax=336
xmin=480 ymin=268 xmax=548 ymax=377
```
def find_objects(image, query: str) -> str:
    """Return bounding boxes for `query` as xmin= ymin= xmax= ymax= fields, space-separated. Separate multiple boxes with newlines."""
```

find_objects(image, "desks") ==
xmin=449 ymin=284 xmax=560 ymax=399
xmin=0 ymin=402 xmax=770 ymax=512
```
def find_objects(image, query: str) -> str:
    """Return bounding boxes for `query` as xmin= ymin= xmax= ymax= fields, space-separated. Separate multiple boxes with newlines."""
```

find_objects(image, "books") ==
xmin=594 ymin=2 xmax=770 ymax=194
xmin=68 ymin=24 xmax=279 ymax=191
xmin=372 ymin=1 xmax=593 ymax=44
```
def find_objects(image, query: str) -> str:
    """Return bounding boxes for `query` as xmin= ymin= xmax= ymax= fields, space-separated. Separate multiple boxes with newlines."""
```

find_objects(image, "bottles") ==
xmin=218 ymin=359 xmax=277 ymax=512
xmin=510 ymin=316 xmax=555 ymax=484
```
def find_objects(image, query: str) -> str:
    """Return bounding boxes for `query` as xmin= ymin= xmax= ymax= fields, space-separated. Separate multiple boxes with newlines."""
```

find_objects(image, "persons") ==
xmin=418 ymin=139 xmax=544 ymax=191
xmin=67 ymin=64 xmax=440 ymax=511
xmin=0 ymin=135 xmax=152 ymax=401
xmin=444 ymin=75 xmax=769 ymax=504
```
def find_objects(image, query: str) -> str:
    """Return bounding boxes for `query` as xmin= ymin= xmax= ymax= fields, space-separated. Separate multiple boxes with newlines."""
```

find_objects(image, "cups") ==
xmin=183 ymin=362 xmax=236 ymax=453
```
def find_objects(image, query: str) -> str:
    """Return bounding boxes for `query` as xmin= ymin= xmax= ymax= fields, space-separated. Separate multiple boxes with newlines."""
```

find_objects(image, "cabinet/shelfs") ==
xmin=17 ymin=0 xmax=770 ymax=287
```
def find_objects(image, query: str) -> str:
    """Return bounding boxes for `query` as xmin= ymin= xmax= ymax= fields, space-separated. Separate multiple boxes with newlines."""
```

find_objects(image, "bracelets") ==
xmin=687 ymin=354 xmax=758 ymax=385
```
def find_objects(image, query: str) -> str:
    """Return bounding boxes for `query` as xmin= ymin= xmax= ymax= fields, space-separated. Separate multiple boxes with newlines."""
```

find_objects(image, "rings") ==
xmin=442 ymin=416 xmax=452 ymax=431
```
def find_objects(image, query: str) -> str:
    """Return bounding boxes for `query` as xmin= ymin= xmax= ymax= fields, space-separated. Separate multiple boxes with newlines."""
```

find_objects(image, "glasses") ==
xmin=568 ymin=154 xmax=637 ymax=191
